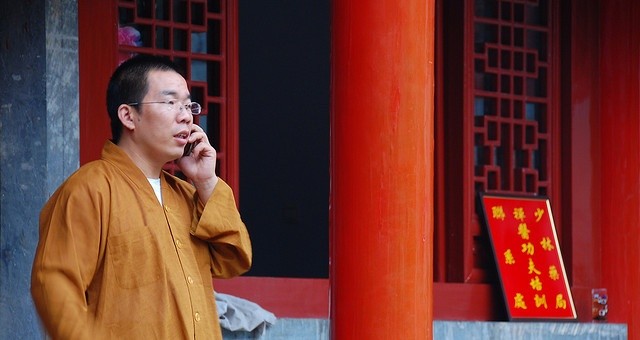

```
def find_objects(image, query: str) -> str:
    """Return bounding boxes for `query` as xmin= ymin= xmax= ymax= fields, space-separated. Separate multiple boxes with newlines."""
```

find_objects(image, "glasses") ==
xmin=128 ymin=98 xmax=202 ymax=115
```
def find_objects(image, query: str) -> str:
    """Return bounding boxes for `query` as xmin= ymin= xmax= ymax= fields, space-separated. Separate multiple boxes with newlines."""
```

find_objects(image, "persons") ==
xmin=29 ymin=52 xmax=253 ymax=340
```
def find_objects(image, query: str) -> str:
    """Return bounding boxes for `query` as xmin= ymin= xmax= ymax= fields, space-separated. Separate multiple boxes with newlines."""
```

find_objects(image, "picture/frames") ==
xmin=479 ymin=190 xmax=579 ymax=322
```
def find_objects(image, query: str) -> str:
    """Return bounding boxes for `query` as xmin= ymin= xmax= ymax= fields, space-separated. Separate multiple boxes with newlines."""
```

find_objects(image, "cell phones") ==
xmin=184 ymin=139 xmax=195 ymax=158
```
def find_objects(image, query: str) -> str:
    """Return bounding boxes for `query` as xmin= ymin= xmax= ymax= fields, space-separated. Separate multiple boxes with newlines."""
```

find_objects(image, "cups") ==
xmin=590 ymin=288 xmax=608 ymax=323
